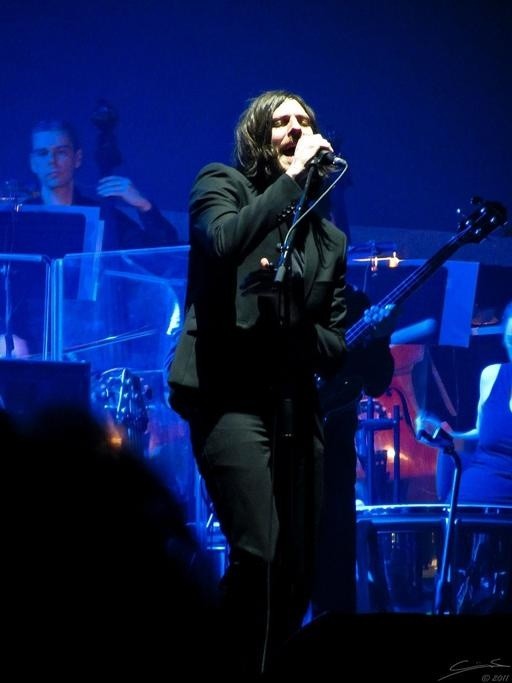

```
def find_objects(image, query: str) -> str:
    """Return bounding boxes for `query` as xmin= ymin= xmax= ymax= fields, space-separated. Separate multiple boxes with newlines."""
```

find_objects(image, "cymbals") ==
xmin=347 ymin=242 xmax=400 ymax=256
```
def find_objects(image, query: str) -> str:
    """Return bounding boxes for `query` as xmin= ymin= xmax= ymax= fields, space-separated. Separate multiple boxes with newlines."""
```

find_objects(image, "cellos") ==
xmin=354 ymin=344 xmax=449 ymax=502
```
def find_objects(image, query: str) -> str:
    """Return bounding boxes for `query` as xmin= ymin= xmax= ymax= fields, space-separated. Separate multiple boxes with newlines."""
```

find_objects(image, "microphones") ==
xmin=317 ymin=148 xmax=347 ymax=166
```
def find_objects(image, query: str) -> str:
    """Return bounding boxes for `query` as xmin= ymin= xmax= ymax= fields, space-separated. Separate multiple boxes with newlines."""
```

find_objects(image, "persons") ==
xmin=1 ymin=118 xmax=185 ymax=363
xmin=0 ymin=399 xmax=240 ymax=681
xmin=170 ymin=86 xmax=346 ymax=626
xmin=307 ymin=286 xmax=394 ymax=619
xmin=415 ymin=293 xmax=512 ymax=502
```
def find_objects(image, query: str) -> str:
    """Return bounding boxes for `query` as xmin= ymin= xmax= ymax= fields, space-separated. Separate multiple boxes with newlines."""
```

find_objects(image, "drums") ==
xmin=356 ymin=503 xmax=512 ymax=615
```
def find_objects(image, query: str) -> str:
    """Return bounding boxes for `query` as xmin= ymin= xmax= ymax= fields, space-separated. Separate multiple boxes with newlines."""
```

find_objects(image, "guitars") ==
xmin=98 ymin=367 xmax=153 ymax=465
xmin=313 ymin=195 xmax=508 ymax=427
